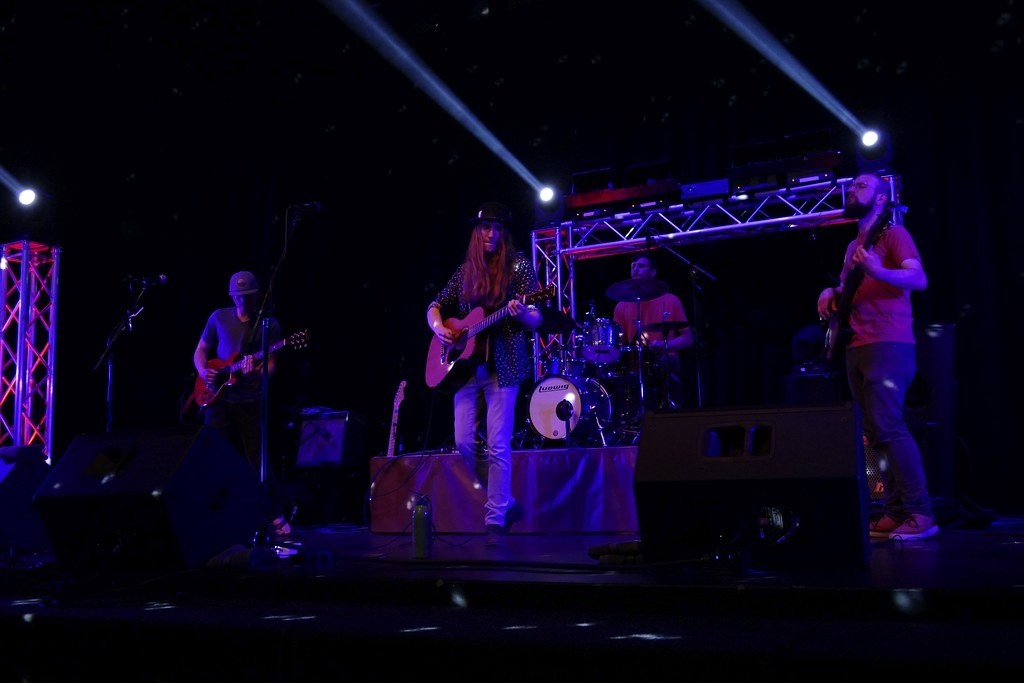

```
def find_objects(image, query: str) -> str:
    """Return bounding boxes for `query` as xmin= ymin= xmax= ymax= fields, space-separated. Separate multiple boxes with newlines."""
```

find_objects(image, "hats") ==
xmin=227 ymin=271 xmax=259 ymax=296
xmin=479 ymin=202 xmax=511 ymax=232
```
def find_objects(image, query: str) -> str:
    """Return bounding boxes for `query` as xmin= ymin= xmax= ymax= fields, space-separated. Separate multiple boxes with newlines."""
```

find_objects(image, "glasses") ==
xmin=847 ymin=182 xmax=880 ymax=192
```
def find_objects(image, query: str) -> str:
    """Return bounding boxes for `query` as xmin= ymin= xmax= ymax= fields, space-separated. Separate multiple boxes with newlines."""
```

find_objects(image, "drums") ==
xmin=547 ymin=357 xmax=586 ymax=377
xmin=526 ymin=373 xmax=612 ymax=442
xmin=579 ymin=318 xmax=625 ymax=365
xmin=604 ymin=345 xmax=652 ymax=384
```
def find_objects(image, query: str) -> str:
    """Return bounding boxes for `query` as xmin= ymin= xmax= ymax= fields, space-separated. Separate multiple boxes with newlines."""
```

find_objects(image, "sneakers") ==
xmin=887 ymin=513 xmax=941 ymax=539
xmin=868 ymin=513 xmax=908 ymax=539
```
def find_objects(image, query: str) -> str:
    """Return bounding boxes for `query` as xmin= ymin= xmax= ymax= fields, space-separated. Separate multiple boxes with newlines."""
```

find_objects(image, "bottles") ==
xmin=411 ymin=500 xmax=432 ymax=559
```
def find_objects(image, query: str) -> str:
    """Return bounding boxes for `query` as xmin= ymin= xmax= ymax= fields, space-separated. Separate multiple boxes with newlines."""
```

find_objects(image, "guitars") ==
xmin=823 ymin=200 xmax=896 ymax=369
xmin=423 ymin=281 xmax=558 ymax=395
xmin=193 ymin=328 xmax=311 ymax=409
xmin=386 ymin=380 xmax=409 ymax=457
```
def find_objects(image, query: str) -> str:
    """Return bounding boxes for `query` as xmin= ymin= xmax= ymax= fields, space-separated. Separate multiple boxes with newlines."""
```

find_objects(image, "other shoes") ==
xmin=484 ymin=521 xmax=505 ymax=548
xmin=504 ymin=499 xmax=527 ymax=533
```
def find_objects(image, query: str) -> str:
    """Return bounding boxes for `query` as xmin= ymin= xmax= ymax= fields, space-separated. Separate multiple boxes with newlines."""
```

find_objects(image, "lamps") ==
xmin=15 ymin=183 xmax=52 ymax=228
xmin=853 ymin=123 xmax=896 ymax=173
xmin=535 ymin=176 xmax=565 ymax=220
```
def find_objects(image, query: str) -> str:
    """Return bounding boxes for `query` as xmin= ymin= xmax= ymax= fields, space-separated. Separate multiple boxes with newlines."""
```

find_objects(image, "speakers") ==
xmin=31 ymin=421 xmax=276 ymax=580
xmin=297 ymin=410 xmax=366 ymax=468
xmin=860 ymin=433 xmax=895 ymax=505
xmin=784 ymin=373 xmax=843 ymax=407
xmin=0 ymin=443 xmax=60 ymax=568
xmin=634 ymin=403 xmax=873 ymax=586
xmin=901 ymin=320 xmax=957 ymax=505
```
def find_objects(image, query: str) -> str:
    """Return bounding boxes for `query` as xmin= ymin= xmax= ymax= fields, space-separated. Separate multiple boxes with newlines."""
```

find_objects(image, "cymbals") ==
xmin=606 ymin=278 xmax=670 ymax=302
xmin=644 ymin=320 xmax=691 ymax=332
xmin=528 ymin=306 xmax=576 ymax=334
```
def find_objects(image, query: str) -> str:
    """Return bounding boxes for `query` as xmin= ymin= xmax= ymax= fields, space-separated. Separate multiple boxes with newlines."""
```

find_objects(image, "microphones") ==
xmin=646 ymin=229 xmax=651 ymax=254
xmin=137 ymin=274 xmax=167 ymax=284
xmin=288 ymin=201 xmax=321 ymax=214
xmin=561 ymin=404 xmax=569 ymax=415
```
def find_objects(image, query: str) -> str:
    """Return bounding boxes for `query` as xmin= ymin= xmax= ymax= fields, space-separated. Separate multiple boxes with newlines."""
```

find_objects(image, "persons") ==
xmin=614 ymin=254 xmax=693 ymax=377
xmin=193 ymin=272 xmax=281 ymax=460
xmin=427 ymin=207 xmax=543 ymax=548
xmin=819 ymin=173 xmax=939 ymax=540
